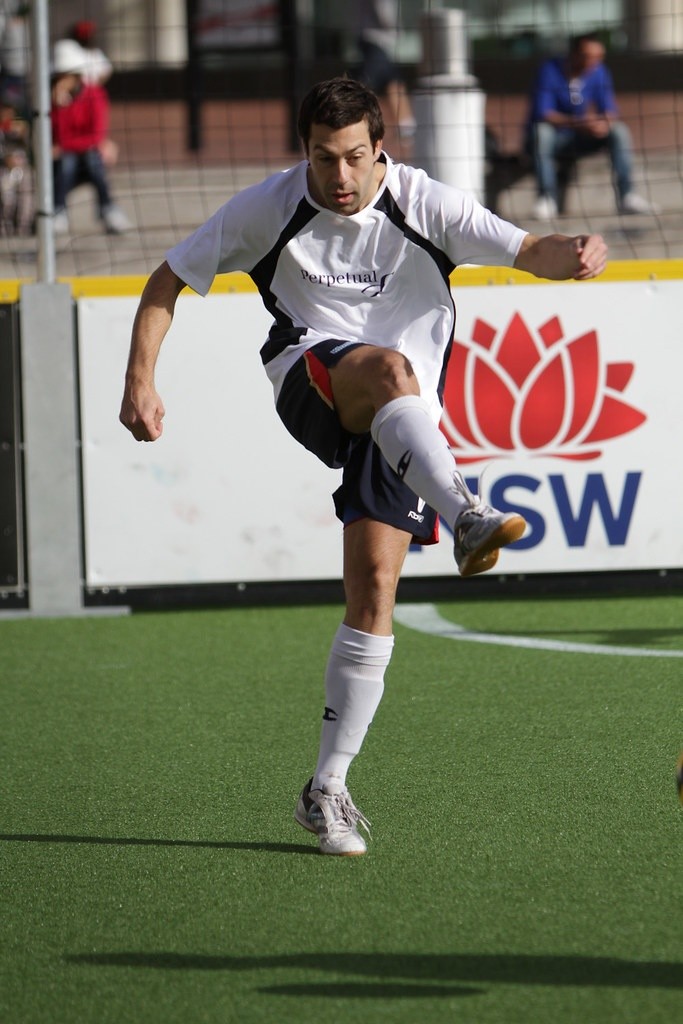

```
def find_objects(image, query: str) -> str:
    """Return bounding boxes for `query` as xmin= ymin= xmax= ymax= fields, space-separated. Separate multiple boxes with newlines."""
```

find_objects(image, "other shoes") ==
xmin=51 ymin=208 xmax=73 ymax=233
xmin=616 ymin=194 xmax=660 ymax=216
xmin=97 ymin=211 xmax=134 ymax=235
xmin=528 ymin=197 xmax=557 ymax=221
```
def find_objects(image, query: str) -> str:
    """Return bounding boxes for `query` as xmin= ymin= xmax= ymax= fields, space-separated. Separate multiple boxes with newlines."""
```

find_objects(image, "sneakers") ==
xmin=293 ymin=776 xmax=373 ymax=857
xmin=453 ymin=469 xmax=527 ymax=579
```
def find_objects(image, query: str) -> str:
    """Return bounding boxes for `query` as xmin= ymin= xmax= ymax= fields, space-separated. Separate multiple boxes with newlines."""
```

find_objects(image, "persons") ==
xmin=120 ymin=74 xmax=609 ymax=856
xmin=0 ymin=0 xmax=131 ymax=233
xmin=309 ymin=0 xmax=418 ymax=136
xmin=527 ymin=36 xmax=659 ymax=221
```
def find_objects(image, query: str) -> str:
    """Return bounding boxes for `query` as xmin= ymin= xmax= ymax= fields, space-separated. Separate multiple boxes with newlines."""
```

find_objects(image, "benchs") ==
xmin=483 ymin=124 xmax=607 ymax=218
